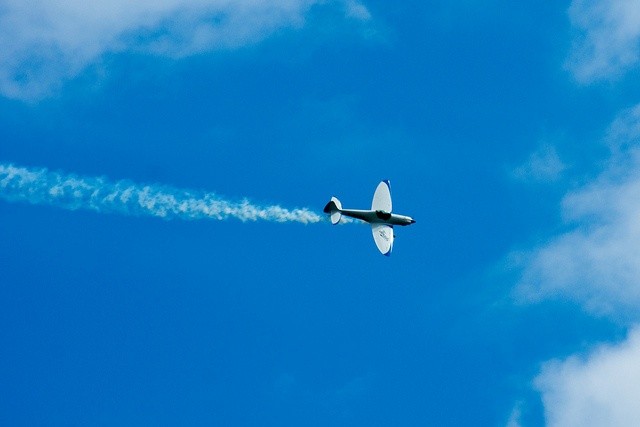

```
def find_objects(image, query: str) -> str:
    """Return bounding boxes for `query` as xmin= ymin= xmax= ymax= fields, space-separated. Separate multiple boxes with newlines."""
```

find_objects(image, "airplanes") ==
xmin=324 ymin=178 xmax=416 ymax=257
